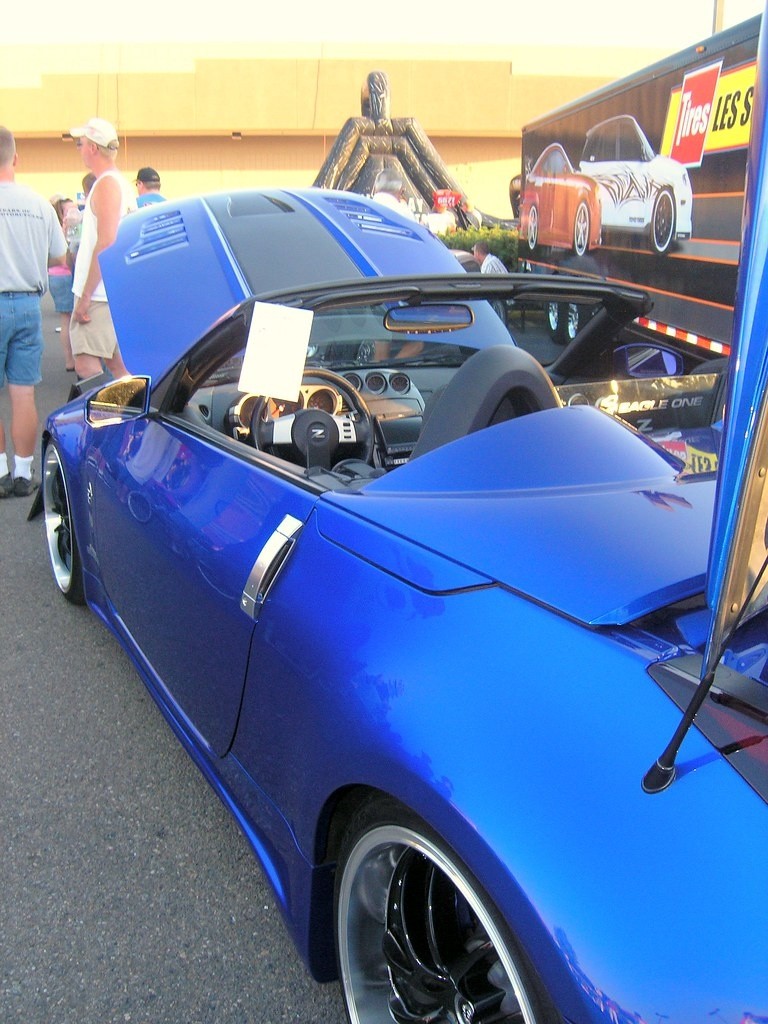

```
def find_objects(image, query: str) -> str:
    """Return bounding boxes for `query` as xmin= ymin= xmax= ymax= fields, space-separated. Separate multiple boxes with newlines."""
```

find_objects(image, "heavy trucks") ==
xmin=523 ymin=11 xmax=762 ymax=364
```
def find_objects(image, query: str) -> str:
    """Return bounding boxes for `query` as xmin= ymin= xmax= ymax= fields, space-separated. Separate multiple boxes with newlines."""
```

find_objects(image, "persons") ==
xmin=0 ymin=129 xmax=68 ymax=498
xmin=474 ymin=243 xmax=507 ymax=275
xmin=47 ymin=119 xmax=132 ymax=385
xmin=135 ymin=169 xmax=168 ymax=207
xmin=370 ymin=169 xmax=416 ymax=222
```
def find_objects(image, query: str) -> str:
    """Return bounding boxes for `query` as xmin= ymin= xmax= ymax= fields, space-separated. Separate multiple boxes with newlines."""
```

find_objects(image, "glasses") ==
xmin=77 ymin=140 xmax=83 ymax=146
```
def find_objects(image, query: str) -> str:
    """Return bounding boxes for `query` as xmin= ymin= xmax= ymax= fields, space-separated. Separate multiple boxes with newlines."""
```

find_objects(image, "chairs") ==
xmin=408 ymin=346 xmax=565 ymax=465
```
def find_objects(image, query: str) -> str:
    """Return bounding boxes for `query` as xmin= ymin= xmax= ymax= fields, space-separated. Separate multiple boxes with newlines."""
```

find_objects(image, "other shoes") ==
xmin=0 ymin=472 xmax=14 ymax=497
xmin=66 ymin=367 xmax=75 ymax=372
xmin=14 ymin=468 xmax=41 ymax=497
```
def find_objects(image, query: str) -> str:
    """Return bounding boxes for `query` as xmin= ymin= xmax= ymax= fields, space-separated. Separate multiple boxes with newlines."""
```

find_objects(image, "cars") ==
xmin=577 ymin=115 xmax=693 ymax=255
xmin=522 ymin=142 xmax=604 ymax=256
xmin=305 ymin=248 xmax=515 ymax=354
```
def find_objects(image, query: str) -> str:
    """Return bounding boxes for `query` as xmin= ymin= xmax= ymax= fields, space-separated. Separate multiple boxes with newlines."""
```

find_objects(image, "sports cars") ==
xmin=28 ymin=2 xmax=768 ymax=1023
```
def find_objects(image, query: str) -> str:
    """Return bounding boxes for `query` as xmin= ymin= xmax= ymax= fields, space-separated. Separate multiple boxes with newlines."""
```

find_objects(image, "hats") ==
xmin=70 ymin=120 xmax=120 ymax=149
xmin=134 ymin=168 xmax=160 ymax=182
